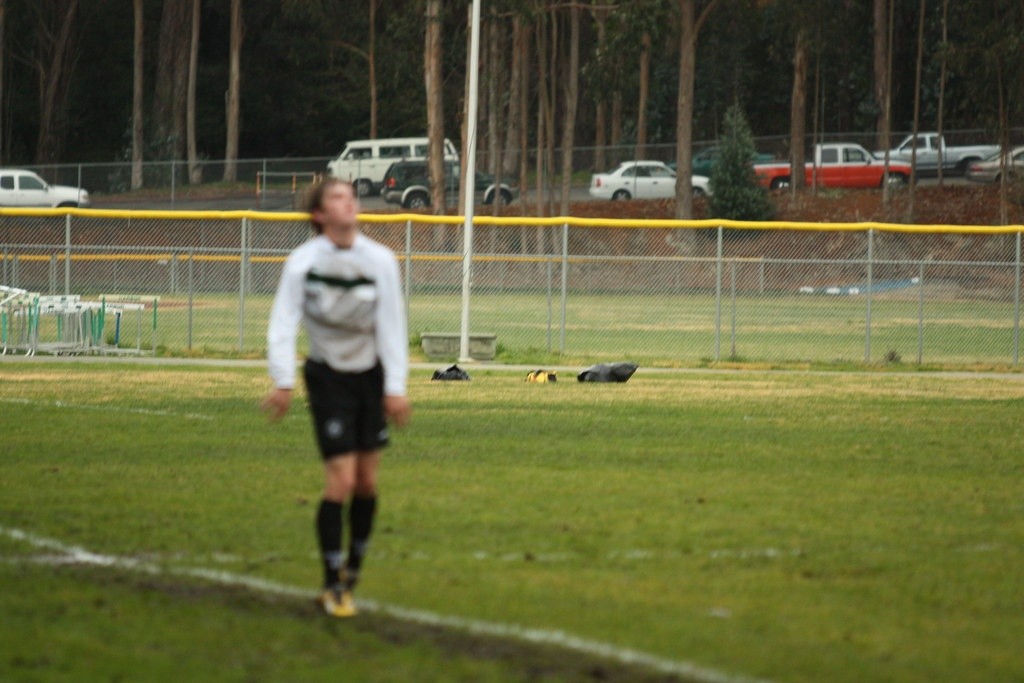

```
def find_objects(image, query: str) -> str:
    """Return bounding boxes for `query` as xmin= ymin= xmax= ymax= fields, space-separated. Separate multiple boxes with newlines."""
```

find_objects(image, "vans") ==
xmin=325 ymin=136 xmax=459 ymax=198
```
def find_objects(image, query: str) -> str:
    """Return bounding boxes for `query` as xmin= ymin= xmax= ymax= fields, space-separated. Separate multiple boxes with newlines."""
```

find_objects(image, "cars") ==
xmin=0 ymin=169 xmax=93 ymax=210
xmin=589 ymin=160 xmax=713 ymax=203
xmin=658 ymin=145 xmax=779 ymax=178
xmin=964 ymin=146 xmax=1024 ymax=183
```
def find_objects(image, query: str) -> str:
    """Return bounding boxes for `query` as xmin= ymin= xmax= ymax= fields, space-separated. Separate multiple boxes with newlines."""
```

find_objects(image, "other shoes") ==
xmin=321 ymin=588 xmax=352 ymax=618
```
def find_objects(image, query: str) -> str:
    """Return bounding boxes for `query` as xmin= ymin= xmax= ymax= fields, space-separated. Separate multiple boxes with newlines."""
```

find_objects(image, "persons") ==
xmin=257 ymin=178 xmax=412 ymax=616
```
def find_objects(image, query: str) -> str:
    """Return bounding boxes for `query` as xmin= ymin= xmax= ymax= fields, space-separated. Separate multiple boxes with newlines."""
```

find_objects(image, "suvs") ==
xmin=379 ymin=160 xmax=521 ymax=209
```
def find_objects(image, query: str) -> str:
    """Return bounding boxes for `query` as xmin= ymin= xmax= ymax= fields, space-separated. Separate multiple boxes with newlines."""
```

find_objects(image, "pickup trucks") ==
xmin=871 ymin=131 xmax=1002 ymax=175
xmin=750 ymin=142 xmax=915 ymax=192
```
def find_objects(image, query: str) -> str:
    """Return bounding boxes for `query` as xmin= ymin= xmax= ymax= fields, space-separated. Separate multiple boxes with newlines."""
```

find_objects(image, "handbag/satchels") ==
xmin=432 ymin=364 xmax=468 ymax=381
xmin=524 ymin=370 xmax=556 ymax=385
